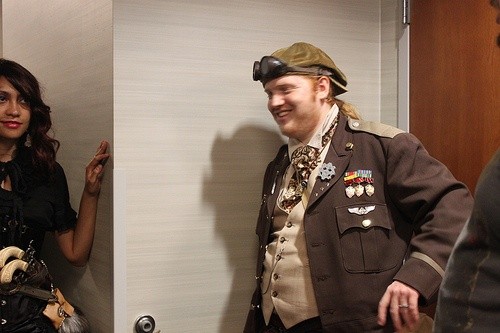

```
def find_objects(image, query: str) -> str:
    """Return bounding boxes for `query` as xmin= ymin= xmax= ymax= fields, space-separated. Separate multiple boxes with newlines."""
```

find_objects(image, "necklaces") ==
xmin=301 ymin=130 xmax=335 ymax=187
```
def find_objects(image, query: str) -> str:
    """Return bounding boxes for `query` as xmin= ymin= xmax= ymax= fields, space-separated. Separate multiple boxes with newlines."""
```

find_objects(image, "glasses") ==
xmin=253 ymin=56 xmax=287 ymax=81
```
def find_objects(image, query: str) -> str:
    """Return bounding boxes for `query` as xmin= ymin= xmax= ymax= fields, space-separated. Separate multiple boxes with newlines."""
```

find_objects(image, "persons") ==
xmin=243 ymin=43 xmax=474 ymax=333
xmin=0 ymin=58 xmax=110 ymax=333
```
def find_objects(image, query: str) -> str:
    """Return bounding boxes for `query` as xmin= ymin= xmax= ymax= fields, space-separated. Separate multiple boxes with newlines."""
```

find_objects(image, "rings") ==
xmin=399 ymin=303 xmax=409 ymax=309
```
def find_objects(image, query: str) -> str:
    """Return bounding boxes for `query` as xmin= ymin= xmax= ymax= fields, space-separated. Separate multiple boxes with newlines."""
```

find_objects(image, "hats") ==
xmin=262 ymin=41 xmax=349 ymax=96
